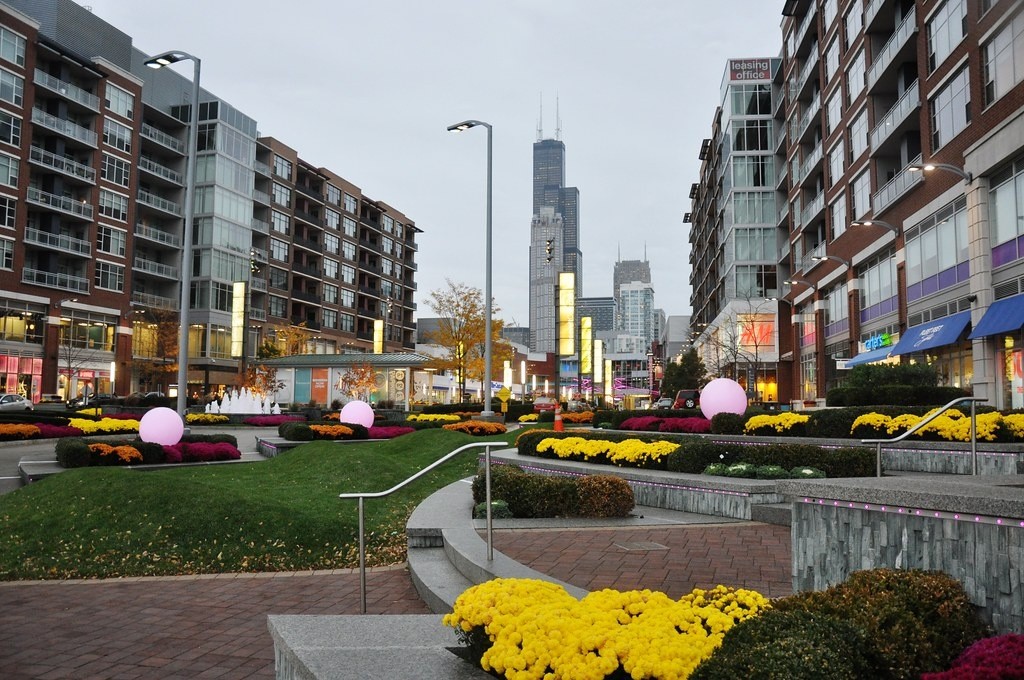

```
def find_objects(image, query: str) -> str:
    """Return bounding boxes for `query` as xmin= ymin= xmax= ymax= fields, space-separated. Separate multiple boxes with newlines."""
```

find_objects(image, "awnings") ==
xmin=890 ymin=310 xmax=971 ymax=356
xmin=967 ymin=293 xmax=1024 ymax=339
xmin=844 ymin=345 xmax=895 ymax=368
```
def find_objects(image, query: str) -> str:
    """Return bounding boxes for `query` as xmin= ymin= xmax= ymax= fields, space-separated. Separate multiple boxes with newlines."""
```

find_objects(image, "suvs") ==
xmin=674 ymin=389 xmax=703 ymax=410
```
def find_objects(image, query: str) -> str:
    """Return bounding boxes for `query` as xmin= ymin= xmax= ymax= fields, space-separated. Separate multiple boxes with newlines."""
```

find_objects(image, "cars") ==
xmin=532 ymin=397 xmax=558 ymax=413
xmin=567 ymin=401 xmax=583 ymax=413
xmin=582 ymin=403 xmax=593 ymax=413
xmin=650 ymin=402 xmax=657 ymax=410
xmin=128 ymin=391 xmax=165 ymax=399
xmin=658 ymin=397 xmax=673 ymax=410
xmin=65 ymin=393 xmax=114 ymax=409
xmin=0 ymin=393 xmax=34 ymax=411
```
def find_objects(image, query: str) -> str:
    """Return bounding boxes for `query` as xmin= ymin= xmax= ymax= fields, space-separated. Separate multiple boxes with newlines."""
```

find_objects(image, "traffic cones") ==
xmin=553 ymin=400 xmax=564 ymax=432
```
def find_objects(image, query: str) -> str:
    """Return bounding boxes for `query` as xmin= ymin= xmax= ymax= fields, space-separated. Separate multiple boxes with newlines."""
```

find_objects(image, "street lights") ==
xmin=447 ymin=117 xmax=497 ymax=416
xmin=142 ymin=50 xmax=201 ymax=423
